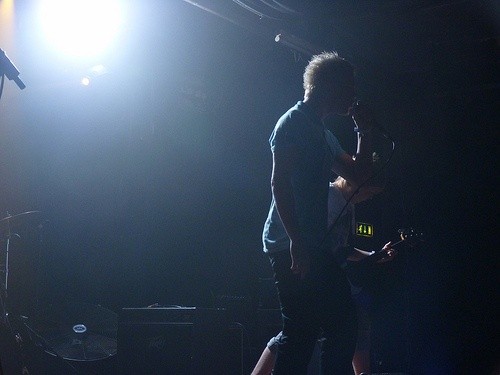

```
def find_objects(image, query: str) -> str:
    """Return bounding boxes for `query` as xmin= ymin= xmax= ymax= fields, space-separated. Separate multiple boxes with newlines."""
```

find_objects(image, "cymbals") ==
xmin=1 ymin=210 xmax=43 ymax=226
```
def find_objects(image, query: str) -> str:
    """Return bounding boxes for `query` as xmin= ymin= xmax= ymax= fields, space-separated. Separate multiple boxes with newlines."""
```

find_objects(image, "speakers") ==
xmin=116 ymin=305 xmax=250 ymax=375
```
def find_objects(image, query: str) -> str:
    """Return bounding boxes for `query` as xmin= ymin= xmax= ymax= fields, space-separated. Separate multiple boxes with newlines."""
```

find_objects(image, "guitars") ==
xmin=345 ymin=225 xmax=427 ymax=289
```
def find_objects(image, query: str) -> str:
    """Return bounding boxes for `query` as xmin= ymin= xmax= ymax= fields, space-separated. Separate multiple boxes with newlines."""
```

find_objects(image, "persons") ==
xmin=261 ymin=51 xmax=374 ymax=375
xmin=249 ymin=152 xmax=397 ymax=375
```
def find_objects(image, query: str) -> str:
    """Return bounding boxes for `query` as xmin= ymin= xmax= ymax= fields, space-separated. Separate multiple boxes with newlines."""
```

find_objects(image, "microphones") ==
xmin=0 ymin=48 xmax=26 ymax=89
